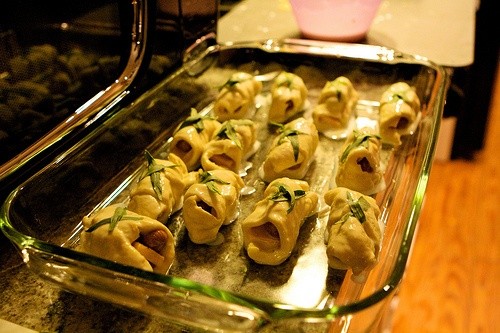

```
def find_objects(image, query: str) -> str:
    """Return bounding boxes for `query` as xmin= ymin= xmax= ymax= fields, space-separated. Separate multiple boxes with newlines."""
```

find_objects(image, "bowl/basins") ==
xmin=291 ymin=0 xmax=382 ymax=40
